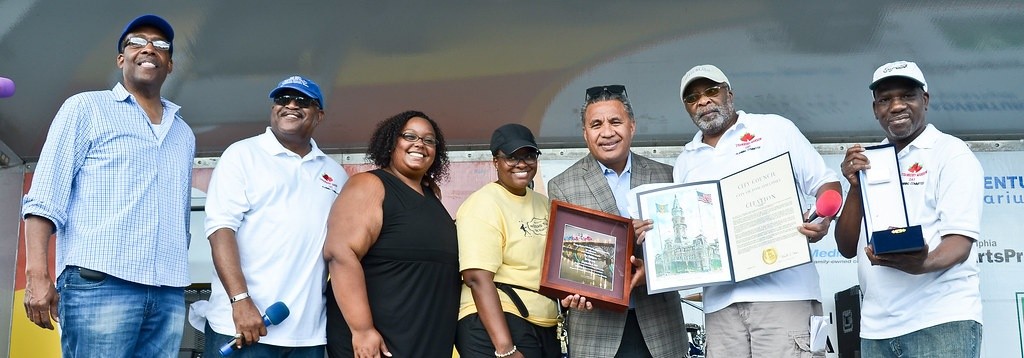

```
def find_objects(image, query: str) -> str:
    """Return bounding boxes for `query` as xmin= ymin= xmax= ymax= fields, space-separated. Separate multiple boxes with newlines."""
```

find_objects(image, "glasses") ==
xmin=272 ymin=94 xmax=321 ymax=110
xmin=584 ymin=85 xmax=627 ymax=101
xmin=495 ymin=150 xmax=538 ymax=165
xmin=124 ymin=38 xmax=171 ymax=53
xmin=398 ymin=132 xmax=441 ymax=147
xmin=683 ymin=85 xmax=729 ymax=104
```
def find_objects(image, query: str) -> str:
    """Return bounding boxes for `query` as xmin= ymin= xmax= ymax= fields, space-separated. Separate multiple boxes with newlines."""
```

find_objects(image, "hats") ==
xmin=679 ymin=64 xmax=731 ymax=106
xmin=269 ymin=76 xmax=323 ymax=113
xmin=490 ymin=123 xmax=541 ymax=153
xmin=117 ymin=14 xmax=174 ymax=57
xmin=869 ymin=61 xmax=928 ymax=100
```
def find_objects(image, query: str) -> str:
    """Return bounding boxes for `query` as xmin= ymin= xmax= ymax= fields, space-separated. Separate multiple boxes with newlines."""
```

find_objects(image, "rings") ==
xmin=850 ymin=159 xmax=853 ymax=165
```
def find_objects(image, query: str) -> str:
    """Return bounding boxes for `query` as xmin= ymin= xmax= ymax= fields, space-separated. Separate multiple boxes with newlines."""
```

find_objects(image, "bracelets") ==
xmin=230 ymin=292 xmax=251 ymax=302
xmin=494 ymin=345 xmax=516 ymax=357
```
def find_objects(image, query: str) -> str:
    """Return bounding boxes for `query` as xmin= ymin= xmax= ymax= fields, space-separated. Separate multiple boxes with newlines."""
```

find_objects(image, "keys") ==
xmin=555 ymin=314 xmax=568 ymax=354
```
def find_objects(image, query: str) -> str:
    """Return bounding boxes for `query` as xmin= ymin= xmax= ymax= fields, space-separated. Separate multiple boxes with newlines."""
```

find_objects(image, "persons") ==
xmin=631 ymin=64 xmax=843 ymax=358
xmin=547 ymin=84 xmax=690 ymax=358
xmin=188 ymin=76 xmax=348 ymax=358
xmin=21 ymin=14 xmax=196 ymax=358
xmin=322 ymin=109 xmax=460 ymax=358
xmin=454 ymin=124 xmax=568 ymax=358
xmin=834 ymin=61 xmax=983 ymax=358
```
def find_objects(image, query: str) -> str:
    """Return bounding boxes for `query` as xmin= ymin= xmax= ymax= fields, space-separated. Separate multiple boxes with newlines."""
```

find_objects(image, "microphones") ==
xmin=0 ymin=76 xmax=16 ymax=98
xmin=217 ymin=301 xmax=290 ymax=356
xmin=807 ymin=190 xmax=843 ymax=223
